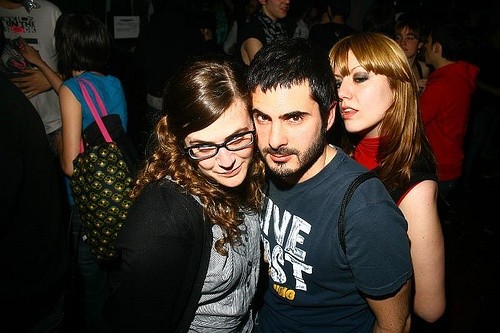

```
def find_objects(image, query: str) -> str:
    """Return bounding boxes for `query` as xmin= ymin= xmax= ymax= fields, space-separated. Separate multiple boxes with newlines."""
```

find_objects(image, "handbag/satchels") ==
xmin=70 ymin=141 xmax=142 ymax=264
xmin=75 ymin=75 xmax=129 ymax=155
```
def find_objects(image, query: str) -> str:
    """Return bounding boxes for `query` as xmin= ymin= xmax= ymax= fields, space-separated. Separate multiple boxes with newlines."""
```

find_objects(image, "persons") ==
xmin=0 ymin=0 xmax=479 ymax=333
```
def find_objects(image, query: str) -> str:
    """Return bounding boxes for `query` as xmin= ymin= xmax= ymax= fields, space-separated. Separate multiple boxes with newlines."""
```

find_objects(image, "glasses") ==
xmin=185 ymin=129 xmax=256 ymax=163
